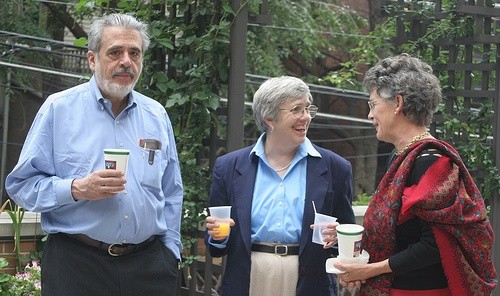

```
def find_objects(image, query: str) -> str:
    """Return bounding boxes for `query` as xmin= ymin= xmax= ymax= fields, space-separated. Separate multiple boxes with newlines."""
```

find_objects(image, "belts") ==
xmin=65 ymin=233 xmax=163 ymax=257
xmin=251 ymin=243 xmax=300 ymax=256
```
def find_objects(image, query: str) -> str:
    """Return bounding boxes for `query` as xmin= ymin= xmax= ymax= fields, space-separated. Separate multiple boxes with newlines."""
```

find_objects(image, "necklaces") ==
xmin=276 ymin=162 xmax=292 ymax=173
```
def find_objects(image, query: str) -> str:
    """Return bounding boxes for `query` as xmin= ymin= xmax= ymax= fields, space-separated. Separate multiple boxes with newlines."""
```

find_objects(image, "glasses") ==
xmin=368 ymin=98 xmax=389 ymax=109
xmin=278 ymin=103 xmax=319 ymax=119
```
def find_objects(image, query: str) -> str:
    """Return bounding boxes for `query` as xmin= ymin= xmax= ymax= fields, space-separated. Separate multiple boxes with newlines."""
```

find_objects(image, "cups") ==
xmin=336 ymin=224 xmax=365 ymax=258
xmin=208 ymin=206 xmax=232 ymax=237
xmin=312 ymin=213 xmax=338 ymax=245
xmin=103 ymin=149 xmax=130 ymax=193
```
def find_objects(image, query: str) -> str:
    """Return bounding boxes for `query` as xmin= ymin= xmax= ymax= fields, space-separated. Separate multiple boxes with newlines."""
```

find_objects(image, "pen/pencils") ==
xmin=139 ymin=139 xmax=146 ymax=159
xmin=148 ymin=148 xmax=155 ymax=165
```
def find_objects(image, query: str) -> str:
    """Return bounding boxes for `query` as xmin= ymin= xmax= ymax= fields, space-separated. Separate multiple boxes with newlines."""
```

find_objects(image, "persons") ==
xmin=334 ymin=53 xmax=498 ymax=296
xmin=203 ymin=75 xmax=356 ymax=296
xmin=5 ymin=13 xmax=184 ymax=296
